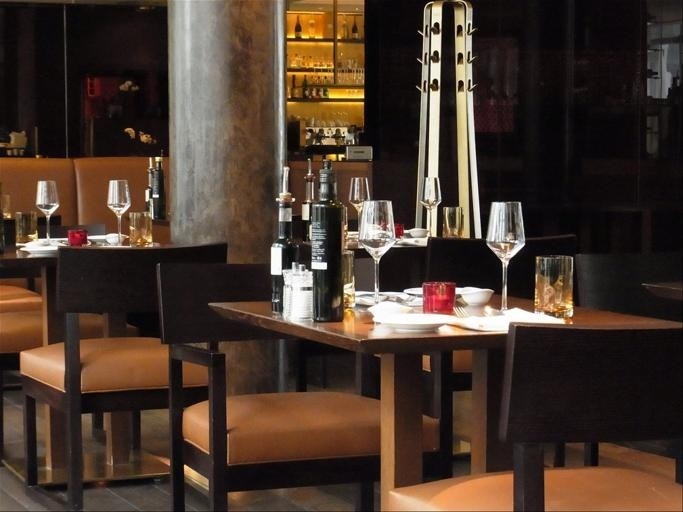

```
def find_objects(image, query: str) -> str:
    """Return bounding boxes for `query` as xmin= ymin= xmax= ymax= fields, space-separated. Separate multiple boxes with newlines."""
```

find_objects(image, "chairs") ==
xmin=20 ymin=238 xmax=230 ymax=512
xmin=3 ymin=157 xmax=376 ymax=467
xmin=387 ymin=322 xmax=683 ymax=511
xmin=419 ymin=236 xmax=682 ymax=477
xmin=153 ymin=259 xmax=438 ymax=511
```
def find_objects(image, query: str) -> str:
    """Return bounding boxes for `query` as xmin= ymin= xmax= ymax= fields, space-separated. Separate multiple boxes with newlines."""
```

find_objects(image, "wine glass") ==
xmin=106 ymin=179 xmax=132 ymax=247
xmin=357 ymin=199 xmax=397 ymax=304
xmin=419 ymin=177 xmax=441 ymax=236
xmin=484 ymin=201 xmax=527 ymax=312
xmin=35 ymin=179 xmax=61 ymax=244
xmin=311 ymin=67 xmax=364 ymax=85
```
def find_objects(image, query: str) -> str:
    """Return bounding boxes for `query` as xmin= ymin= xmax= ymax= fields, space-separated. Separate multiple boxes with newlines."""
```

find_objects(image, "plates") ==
xmin=16 ymin=237 xmax=65 ymax=252
xmin=403 ymin=237 xmax=427 ymax=247
xmin=86 ymin=234 xmax=129 ymax=245
xmin=372 ymin=313 xmax=453 ymax=332
xmin=404 ymin=285 xmax=464 ymax=297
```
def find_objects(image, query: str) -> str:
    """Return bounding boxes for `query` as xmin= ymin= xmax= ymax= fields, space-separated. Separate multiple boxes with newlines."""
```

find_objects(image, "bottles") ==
xmin=281 ymin=261 xmax=312 ymax=320
xmin=294 ymin=14 xmax=302 ymax=38
xmin=290 ymin=73 xmax=330 ymax=99
xmin=143 ymin=155 xmax=168 ymax=219
xmin=269 ymin=191 xmax=301 ymax=314
xmin=300 ymin=172 xmax=317 ymax=242
xmin=307 ymin=10 xmax=316 ymax=38
xmin=310 ymin=158 xmax=347 ymax=325
xmin=351 ymin=14 xmax=358 ymax=39
xmin=341 ymin=19 xmax=349 ymax=38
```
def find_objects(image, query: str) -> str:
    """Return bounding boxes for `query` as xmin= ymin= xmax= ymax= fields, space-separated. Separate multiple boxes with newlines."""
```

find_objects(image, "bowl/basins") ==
xmin=460 ymin=288 xmax=496 ymax=306
xmin=408 ymin=227 xmax=428 ymax=238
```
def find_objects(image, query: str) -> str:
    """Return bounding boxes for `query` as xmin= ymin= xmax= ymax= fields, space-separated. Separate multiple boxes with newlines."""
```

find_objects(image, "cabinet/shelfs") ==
xmin=285 ymin=0 xmax=365 ymax=102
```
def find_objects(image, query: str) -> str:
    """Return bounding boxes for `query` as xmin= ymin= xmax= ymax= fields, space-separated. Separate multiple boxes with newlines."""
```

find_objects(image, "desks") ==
xmin=203 ymin=286 xmax=682 ymax=512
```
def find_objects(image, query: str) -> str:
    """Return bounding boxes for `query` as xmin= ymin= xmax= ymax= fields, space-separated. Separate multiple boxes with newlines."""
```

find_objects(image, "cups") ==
xmin=341 ymin=250 xmax=356 ymax=308
xmin=304 ymin=117 xmax=345 ymax=127
xmin=348 ymin=177 xmax=370 ymax=211
xmin=382 ymin=223 xmax=404 ymax=240
xmin=441 ymin=206 xmax=464 ymax=237
xmin=421 ymin=280 xmax=457 ymax=315
xmin=534 ymin=252 xmax=576 ymax=319
xmin=287 ymin=52 xmax=358 ymax=68
xmin=128 ymin=211 xmax=154 ymax=247
xmin=67 ymin=229 xmax=92 ymax=247
xmin=1 ymin=193 xmax=13 ymax=218
xmin=14 ymin=211 xmax=38 ymax=243
xmin=9 ymin=131 xmax=27 ymax=146
